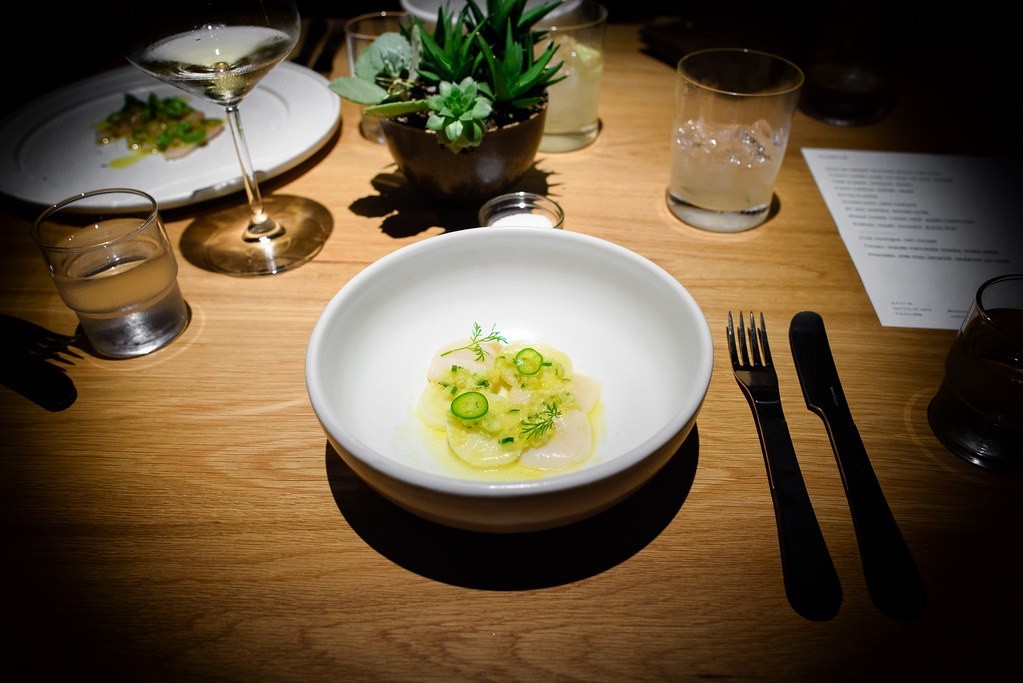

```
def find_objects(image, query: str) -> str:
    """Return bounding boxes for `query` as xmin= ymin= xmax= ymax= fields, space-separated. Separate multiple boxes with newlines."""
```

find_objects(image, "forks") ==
xmin=0 ymin=312 xmax=82 ymax=359
xmin=725 ymin=311 xmax=845 ymax=620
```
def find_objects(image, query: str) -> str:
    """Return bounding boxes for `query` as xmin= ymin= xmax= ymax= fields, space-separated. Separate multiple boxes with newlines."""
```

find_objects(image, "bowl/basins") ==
xmin=306 ymin=226 xmax=716 ymax=536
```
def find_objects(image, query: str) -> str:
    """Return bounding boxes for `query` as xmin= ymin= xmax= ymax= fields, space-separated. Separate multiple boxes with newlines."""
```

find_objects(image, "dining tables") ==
xmin=0 ymin=0 xmax=1023 ymax=683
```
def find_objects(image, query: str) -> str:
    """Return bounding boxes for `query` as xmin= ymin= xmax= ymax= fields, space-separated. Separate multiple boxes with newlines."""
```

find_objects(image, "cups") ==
xmin=666 ymin=47 xmax=804 ymax=233
xmin=34 ymin=189 xmax=191 ymax=360
xmin=926 ymin=275 xmax=1023 ymax=475
xmin=519 ymin=4 xmax=608 ymax=150
xmin=346 ymin=13 xmax=434 ymax=146
xmin=480 ymin=191 xmax=564 ymax=230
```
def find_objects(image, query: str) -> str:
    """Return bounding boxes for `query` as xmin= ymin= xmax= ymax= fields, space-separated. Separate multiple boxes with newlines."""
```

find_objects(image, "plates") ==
xmin=0 ymin=62 xmax=342 ymax=212
xmin=401 ymin=0 xmax=583 ymax=32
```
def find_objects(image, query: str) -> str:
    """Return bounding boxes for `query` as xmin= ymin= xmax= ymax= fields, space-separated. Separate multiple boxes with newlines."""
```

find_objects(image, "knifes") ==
xmin=788 ymin=311 xmax=926 ymax=619
xmin=0 ymin=348 xmax=77 ymax=412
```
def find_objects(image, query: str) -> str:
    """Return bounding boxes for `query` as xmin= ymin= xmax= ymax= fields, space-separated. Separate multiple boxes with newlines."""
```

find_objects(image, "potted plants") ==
xmin=328 ymin=0 xmax=569 ymax=202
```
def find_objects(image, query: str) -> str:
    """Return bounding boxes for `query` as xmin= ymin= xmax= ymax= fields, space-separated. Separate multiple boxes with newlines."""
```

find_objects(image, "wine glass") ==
xmin=110 ymin=0 xmax=335 ymax=279
